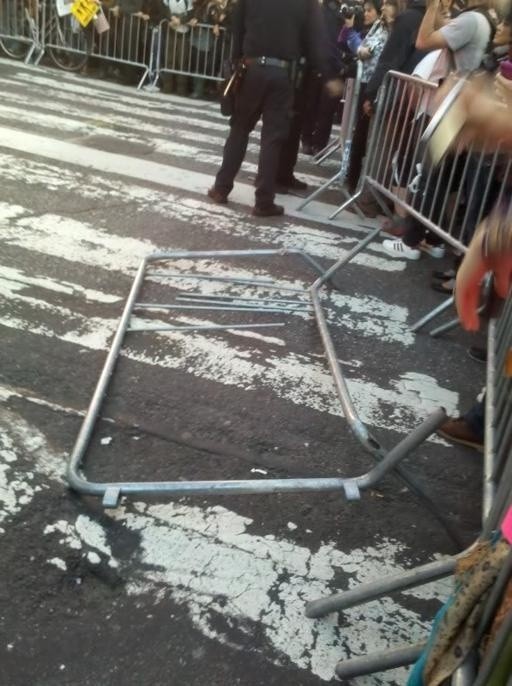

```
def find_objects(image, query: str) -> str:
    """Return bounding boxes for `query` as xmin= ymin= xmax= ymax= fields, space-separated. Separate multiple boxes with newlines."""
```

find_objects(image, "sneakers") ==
xmin=429 ymin=268 xmax=457 ymax=294
xmin=433 ymin=413 xmax=488 ymax=454
xmin=419 ymin=238 xmax=446 ymax=260
xmin=382 ymin=237 xmax=421 ymax=261
xmin=287 ymin=176 xmax=308 ymax=190
xmin=251 ymin=204 xmax=284 ymax=217
xmin=466 ymin=345 xmax=487 ymax=364
xmin=206 ymin=183 xmax=230 ymax=203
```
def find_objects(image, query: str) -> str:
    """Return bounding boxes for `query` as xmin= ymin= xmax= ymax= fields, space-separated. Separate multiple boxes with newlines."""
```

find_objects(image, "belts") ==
xmin=245 ymin=55 xmax=295 ymax=70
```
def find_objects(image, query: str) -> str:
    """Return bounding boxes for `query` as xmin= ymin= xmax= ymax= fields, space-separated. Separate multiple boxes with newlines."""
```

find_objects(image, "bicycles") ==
xmin=0 ymin=0 xmax=92 ymax=71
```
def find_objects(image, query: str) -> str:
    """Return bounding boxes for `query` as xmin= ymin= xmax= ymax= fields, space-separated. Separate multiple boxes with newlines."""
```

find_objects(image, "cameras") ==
xmin=343 ymin=6 xmax=365 ymax=23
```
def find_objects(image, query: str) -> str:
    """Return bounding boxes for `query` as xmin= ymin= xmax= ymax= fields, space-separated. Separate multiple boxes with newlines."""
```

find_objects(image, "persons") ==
xmin=2 ymin=1 xmax=228 ymax=100
xmin=209 ymin=1 xmax=341 ymax=216
xmin=342 ymin=2 xmax=510 ymax=542
